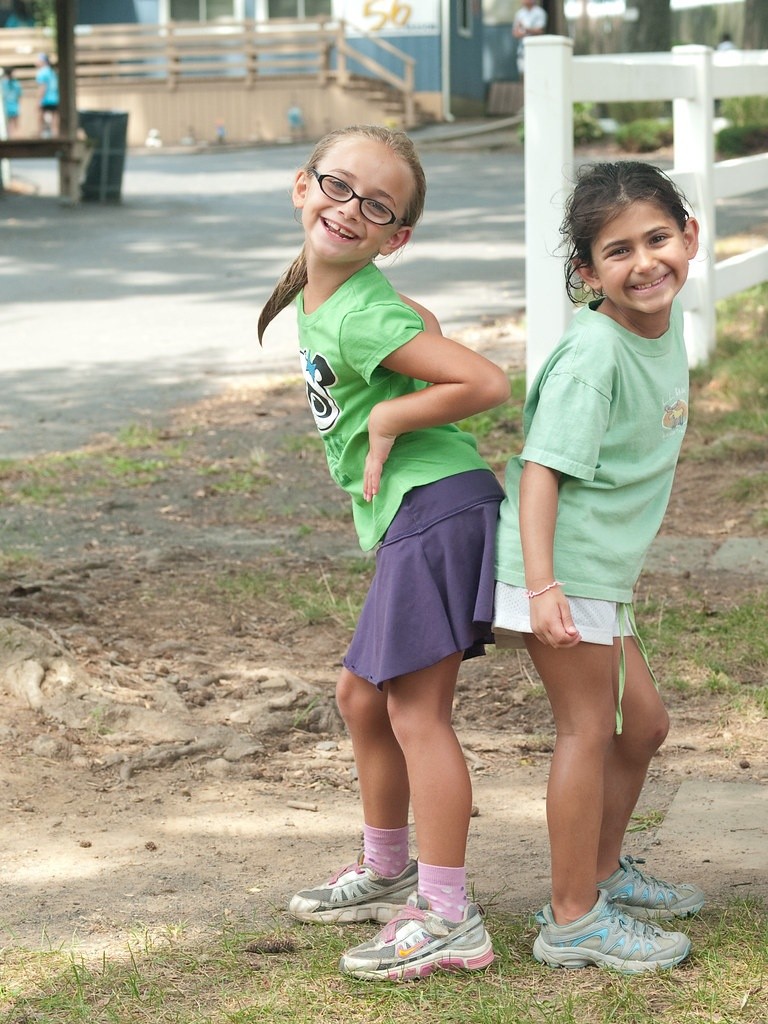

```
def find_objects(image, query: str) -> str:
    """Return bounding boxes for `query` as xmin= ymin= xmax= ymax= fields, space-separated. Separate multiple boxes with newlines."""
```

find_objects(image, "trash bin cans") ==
xmin=77 ymin=109 xmax=129 ymax=203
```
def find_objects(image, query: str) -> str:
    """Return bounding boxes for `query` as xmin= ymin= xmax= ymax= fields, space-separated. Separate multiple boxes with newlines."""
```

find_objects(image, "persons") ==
xmin=0 ymin=0 xmax=59 ymax=140
xmin=717 ymin=33 xmax=738 ymax=50
xmin=512 ymin=0 xmax=547 ymax=82
xmin=258 ymin=127 xmax=514 ymax=982
xmin=495 ymin=159 xmax=701 ymax=974
xmin=289 ymin=100 xmax=305 ymax=145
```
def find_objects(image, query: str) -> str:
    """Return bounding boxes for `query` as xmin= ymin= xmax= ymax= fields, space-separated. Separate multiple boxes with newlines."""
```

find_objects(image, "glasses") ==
xmin=311 ymin=168 xmax=404 ymax=226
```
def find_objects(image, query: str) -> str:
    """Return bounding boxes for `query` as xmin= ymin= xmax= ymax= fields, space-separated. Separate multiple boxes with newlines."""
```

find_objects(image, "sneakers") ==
xmin=596 ymin=856 xmax=704 ymax=920
xmin=532 ymin=889 xmax=691 ymax=974
xmin=290 ymin=850 xmax=418 ymax=925
xmin=338 ymin=890 xmax=494 ymax=981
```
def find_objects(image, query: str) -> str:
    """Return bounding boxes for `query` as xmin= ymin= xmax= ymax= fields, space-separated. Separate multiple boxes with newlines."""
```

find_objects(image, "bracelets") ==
xmin=524 ymin=582 xmax=560 ymax=599
